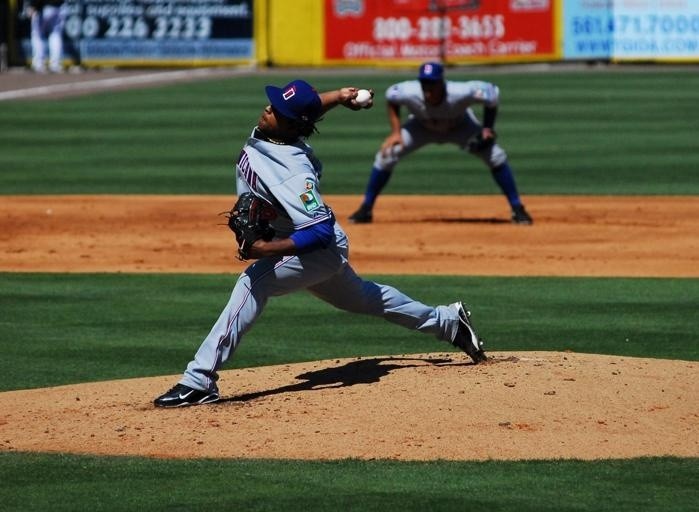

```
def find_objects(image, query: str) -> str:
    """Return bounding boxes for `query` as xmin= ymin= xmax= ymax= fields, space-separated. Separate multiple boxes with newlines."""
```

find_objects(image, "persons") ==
xmin=21 ymin=0 xmax=67 ymax=74
xmin=155 ymin=79 xmax=488 ymax=408
xmin=347 ymin=62 xmax=531 ymax=224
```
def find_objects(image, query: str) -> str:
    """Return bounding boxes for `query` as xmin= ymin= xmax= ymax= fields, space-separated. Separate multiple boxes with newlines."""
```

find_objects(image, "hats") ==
xmin=418 ymin=62 xmax=443 ymax=80
xmin=265 ymin=79 xmax=323 ymax=125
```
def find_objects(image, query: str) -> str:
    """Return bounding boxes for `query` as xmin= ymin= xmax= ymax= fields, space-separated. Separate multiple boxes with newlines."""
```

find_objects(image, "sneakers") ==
xmin=346 ymin=211 xmax=373 ymax=223
xmin=154 ymin=382 xmax=220 ymax=408
xmin=452 ymin=301 xmax=487 ymax=364
xmin=512 ymin=205 xmax=532 ymax=225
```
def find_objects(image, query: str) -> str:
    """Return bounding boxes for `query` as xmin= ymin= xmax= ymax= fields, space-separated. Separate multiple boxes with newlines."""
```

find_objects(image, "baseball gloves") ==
xmin=465 ymin=127 xmax=496 ymax=154
xmin=217 ymin=192 xmax=277 ymax=262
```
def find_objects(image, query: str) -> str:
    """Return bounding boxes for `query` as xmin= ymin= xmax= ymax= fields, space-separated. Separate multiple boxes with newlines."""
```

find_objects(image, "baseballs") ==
xmin=356 ymin=89 xmax=372 ymax=107
